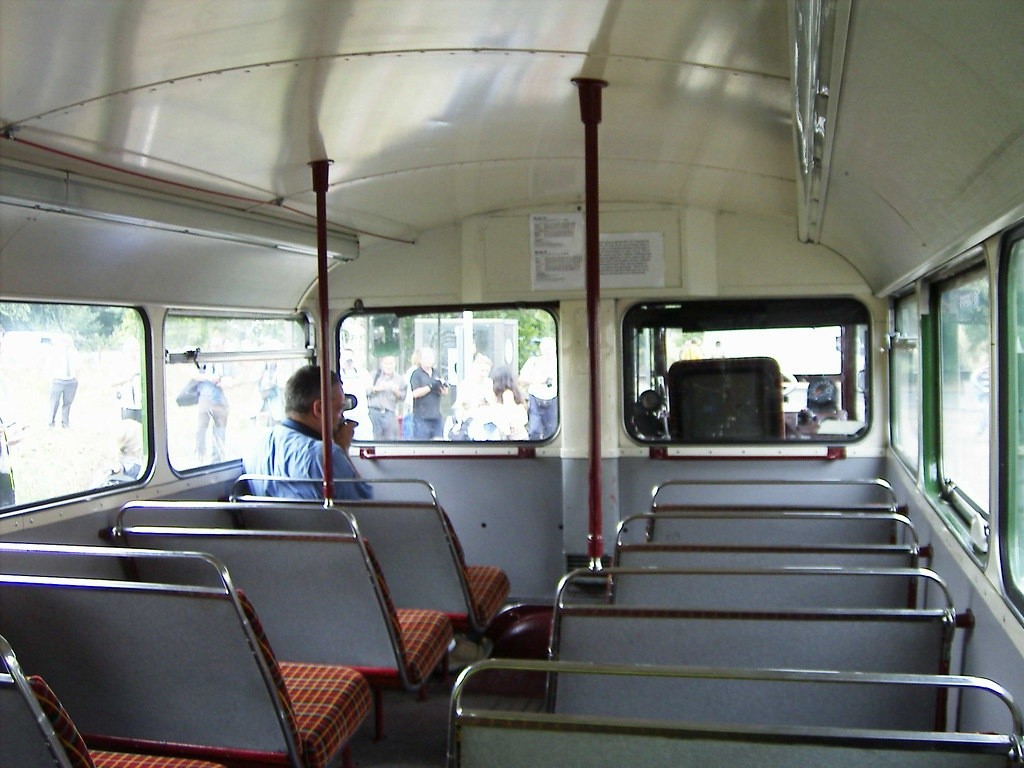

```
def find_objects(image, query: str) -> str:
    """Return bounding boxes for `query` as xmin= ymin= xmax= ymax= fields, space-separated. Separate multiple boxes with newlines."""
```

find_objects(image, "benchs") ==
xmin=0 ymin=474 xmax=1024 ymax=768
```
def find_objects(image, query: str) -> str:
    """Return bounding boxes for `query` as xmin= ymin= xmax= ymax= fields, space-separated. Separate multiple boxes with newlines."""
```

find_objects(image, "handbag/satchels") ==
xmin=176 ymin=368 xmax=205 ymax=405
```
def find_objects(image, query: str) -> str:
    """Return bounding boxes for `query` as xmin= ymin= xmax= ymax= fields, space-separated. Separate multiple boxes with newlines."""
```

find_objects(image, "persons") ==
xmin=242 ymin=365 xmax=374 ymax=501
xmin=192 ymin=329 xmax=237 ymax=467
xmin=341 ymin=333 xmax=559 ymax=441
